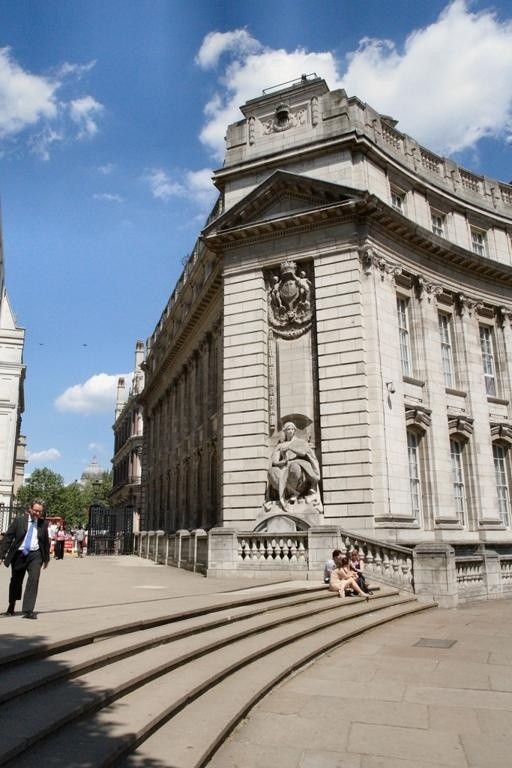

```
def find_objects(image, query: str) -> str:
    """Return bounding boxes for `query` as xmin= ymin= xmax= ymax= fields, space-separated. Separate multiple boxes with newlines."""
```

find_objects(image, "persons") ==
xmin=329 ymin=557 xmax=370 ymax=597
xmin=44 ymin=520 xmax=54 ymax=561
xmin=75 ymin=526 xmax=85 ymax=558
xmin=324 ymin=550 xmax=342 ymax=582
xmin=270 ymin=269 xmax=312 ymax=317
xmin=267 ymin=422 xmax=320 ymax=503
xmin=70 ymin=526 xmax=88 ymax=547
xmin=350 ymin=550 xmax=373 ymax=595
xmin=56 ymin=525 xmax=66 ymax=560
xmin=0 ymin=498 xmax=50 ymax=619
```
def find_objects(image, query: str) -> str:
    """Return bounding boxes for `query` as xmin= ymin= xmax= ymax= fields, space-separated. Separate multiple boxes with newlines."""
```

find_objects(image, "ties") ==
xmin=22 ymin=520 xmax=35 ymax=556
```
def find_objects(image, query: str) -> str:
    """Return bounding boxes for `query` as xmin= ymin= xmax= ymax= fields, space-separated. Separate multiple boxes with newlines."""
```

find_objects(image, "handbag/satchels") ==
xmin=81 ymin=540 xmax=86 ymax=547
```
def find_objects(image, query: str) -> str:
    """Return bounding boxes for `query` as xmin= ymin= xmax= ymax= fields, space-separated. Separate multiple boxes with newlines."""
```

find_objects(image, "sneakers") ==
xmin=6 ymin=603 xmax=37 ymax=619
xmin=358 ymin=592 xmax=370 ymax=598
